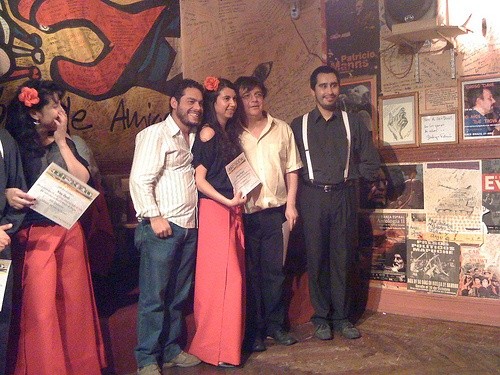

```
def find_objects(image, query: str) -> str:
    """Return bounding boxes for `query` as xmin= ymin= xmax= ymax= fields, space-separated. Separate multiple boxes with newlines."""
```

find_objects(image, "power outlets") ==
xmin=290 ymin=0 xmax=301 ymax=19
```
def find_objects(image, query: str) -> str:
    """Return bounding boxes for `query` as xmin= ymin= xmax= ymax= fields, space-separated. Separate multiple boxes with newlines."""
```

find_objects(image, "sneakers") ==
xmin=137 ymin=364 xmax=161 ymax=375
xmin=334 ymin=319 xmax=360 ymax=339
xmin=314 ymin=321 xmax=332 ymax=339
xmin=163 ymin=350 xmax=201 ymax=368
xmin=264 ymin=330 xmax=298 ymax=346
xmin=246 ymin=333 xmax=266 ymax=351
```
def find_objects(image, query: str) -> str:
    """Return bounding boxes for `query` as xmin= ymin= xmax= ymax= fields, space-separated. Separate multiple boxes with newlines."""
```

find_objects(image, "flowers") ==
xmin=18 ymin=86 xmax=40 ymax=107
xmin=204 ymin=77 xmax=220 ymax=91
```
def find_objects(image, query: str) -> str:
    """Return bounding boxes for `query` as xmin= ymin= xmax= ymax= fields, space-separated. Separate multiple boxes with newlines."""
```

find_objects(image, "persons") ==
xmin=128 ymin=78 xmax=205 ymax=375
xmin=459 ymin=263 xmax=500 ymax=299
xmin=5 ymin=79 xmax=108 ymax=375
xmin=191 ymin=76 xmax=248 ymax=368
xmin=233 ymin=76 xmax=304 ymax=351
xmin=0 ymin=128 xmax=28 ymax=375
xmin=464 ymin=88 xmax=500 ymax=137
xmin=290 ymin=66 xmax=382 ymax=341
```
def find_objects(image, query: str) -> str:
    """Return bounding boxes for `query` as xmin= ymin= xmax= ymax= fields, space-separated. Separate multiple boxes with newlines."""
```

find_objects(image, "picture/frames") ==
xmin=418 ymin=111 xmax=459 ymax=146
xmin=456 ymin=74 xmax=500 ymax=145
xmin=377 ymin=91 xmax=420 ymax=150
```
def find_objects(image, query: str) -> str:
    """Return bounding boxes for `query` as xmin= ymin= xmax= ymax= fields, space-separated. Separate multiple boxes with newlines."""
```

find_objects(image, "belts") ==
xmin=304 ymin=182 xmax=352 ymax=192
